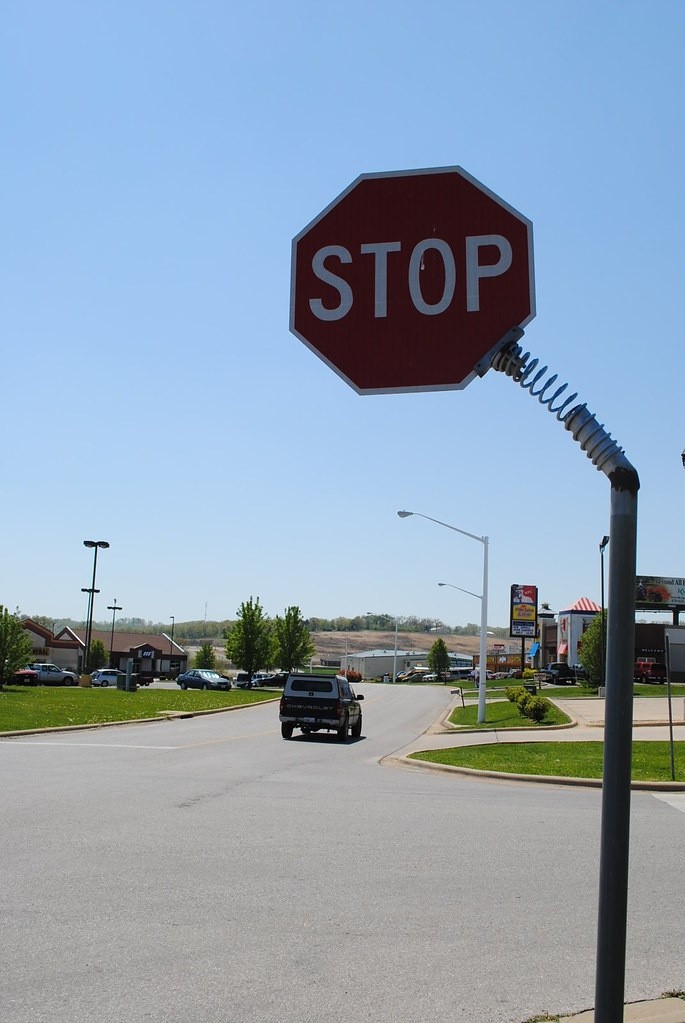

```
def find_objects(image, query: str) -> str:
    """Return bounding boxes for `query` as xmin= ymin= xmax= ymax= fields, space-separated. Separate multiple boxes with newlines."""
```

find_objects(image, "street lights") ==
xmin=367 ymin=611 xmax=397 ymax=684
xmin=79 ymin=588 xmax=100 ymax=671
xmin=82 ymin=540 xmax=109 ymax=674
xmin=106 ymin=598 xmax=122 ymax=665
xmin=169 ymin=616 xmax=175 ymax=666
xmin=598 ymin=535 xmax=610 ymax=687
xmin=398 ymin=510 xmax=489 ymax=721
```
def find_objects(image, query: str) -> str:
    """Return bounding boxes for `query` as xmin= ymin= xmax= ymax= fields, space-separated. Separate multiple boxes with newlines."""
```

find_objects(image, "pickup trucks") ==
xmin=545 ymin=661 xmax=576 ymax=684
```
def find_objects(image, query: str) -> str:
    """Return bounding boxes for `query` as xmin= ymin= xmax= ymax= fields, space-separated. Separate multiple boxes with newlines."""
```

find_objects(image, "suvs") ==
xmin=633 ymin=662 xmax=668 ymax=685
xmin=31 ymin=664 xmax=77 ymax=687
xmin=278 ymin=673 xmax=365 ymax=741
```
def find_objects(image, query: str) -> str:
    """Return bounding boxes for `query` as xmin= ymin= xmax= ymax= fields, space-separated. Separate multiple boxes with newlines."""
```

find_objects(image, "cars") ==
xmin=176 ymin=669 xmax=229 ymax=691
xmin=130 ymin=673 xmax=154 ymax=686
xmin=254 ymin=672 xmax=290 ymax=688
xmin=396 ymin=667 xmax=545 ymax=682
xmin=91 ymin=669 xmax=126 ymax=687
xmin=10 ymin=663 xmax=38 ymax=686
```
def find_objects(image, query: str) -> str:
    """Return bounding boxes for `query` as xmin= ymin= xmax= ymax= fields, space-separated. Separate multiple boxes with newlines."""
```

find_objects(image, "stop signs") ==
xmin=288 ymin=165 xmax=536 ymax=397
